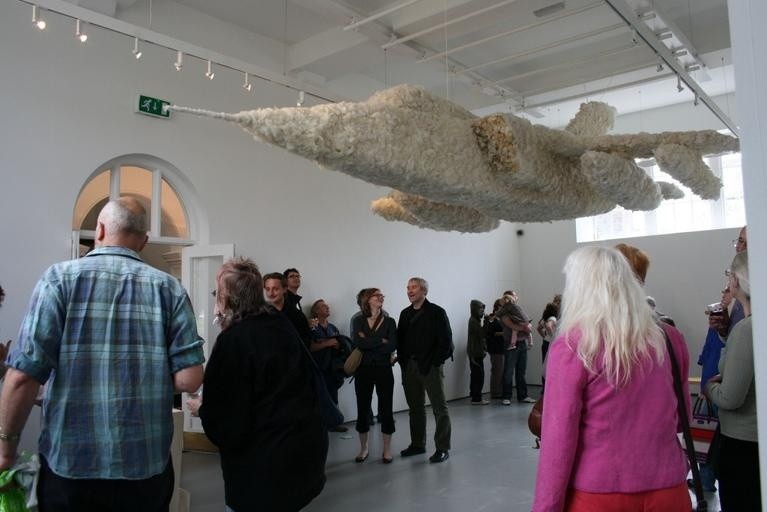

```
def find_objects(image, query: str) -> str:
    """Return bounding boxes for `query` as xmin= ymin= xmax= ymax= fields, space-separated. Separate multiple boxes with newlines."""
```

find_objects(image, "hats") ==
xmin=503 ymin=291 xmax=516 ymax=303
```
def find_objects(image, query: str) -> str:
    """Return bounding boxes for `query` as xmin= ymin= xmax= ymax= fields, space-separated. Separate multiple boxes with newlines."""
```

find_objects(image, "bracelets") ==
xmin=1 ymin=435 xmax=20 ymax=440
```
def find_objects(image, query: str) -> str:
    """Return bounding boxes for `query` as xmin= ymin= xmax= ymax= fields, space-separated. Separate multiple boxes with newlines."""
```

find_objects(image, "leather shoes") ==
xmin=383 ymin=452 xmax=393 ymax=463
xmin=401 ymin=444 xmax=425 ymax=456
xmin=430 ymin=450 xmax=449 ymax=463
xmin=355 ymin=450 xmax=369 ymax=463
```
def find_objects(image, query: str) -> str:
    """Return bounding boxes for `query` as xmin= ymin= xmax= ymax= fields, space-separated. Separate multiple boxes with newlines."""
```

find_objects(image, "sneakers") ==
xmin=503 ymin=399 xmax=511 ymax=405
xmin=328 ymin=426 xmax=349 ymax=433
xmin=518 ymin=397 xmax=537 ymax=403
xmin=471 ymin=399 xmax=491 ymax=405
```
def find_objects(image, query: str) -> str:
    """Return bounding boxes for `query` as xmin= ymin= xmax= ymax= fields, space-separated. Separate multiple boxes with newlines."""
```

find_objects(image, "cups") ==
xmin=184 ymin=389 xmax=201 ymax=417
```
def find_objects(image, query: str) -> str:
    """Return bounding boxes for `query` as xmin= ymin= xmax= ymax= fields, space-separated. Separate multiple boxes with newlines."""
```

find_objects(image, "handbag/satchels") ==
xmin=343 ymin=347 xmax=364 ymax=375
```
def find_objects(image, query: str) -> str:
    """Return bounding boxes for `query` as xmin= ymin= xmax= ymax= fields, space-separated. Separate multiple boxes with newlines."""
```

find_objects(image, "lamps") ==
xmin=629 ymin=13 xmax=701 ymax=107
xmin=31 ymin=1 xmax=306 ymax=109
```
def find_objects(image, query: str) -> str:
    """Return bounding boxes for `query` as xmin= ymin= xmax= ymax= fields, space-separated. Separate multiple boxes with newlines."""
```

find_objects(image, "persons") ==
xmin=397 ymin=277 xmax=456 ymax=462
xmin=261 ymin=268 xmax=311 ymax=353
xmin=0 ymin=195 xmax=204 ymax=511
xmin=308 ymin=299 xmax=348 ymax=433
xmin=697 ymin=227 xmax=762 ymax=512
xmin=467 ymin=291 xmax=537 ymax=406
xmin=186 ymin=256 xmax=329 ymax=512
xmin=0 ymin=289 xmax=44 ymax=407
xmin=531 ymin=242 xmax=694 ymax=512
xmin=349 ymin=288 xmax=398 ymax=464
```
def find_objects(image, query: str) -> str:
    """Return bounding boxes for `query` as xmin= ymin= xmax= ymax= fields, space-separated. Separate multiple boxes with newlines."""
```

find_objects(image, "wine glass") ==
xmin=708 ymin=302 xmax=729 ymax=329
